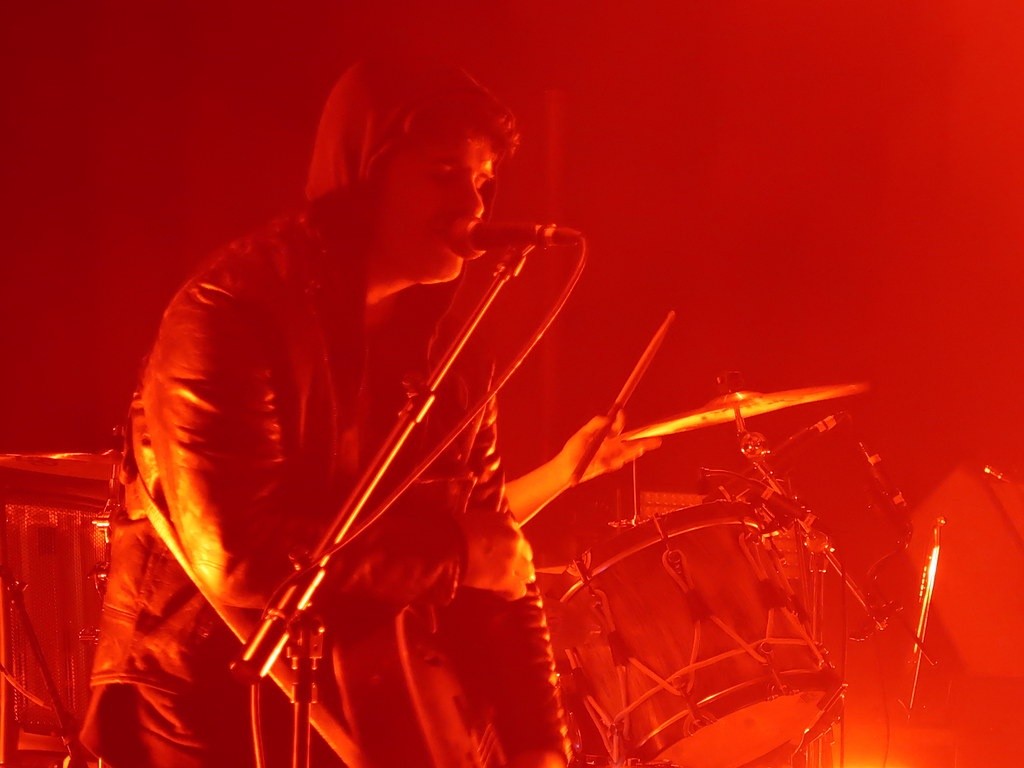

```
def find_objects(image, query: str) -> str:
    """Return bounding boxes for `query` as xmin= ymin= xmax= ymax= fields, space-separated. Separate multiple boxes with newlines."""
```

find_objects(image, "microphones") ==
xmin=859 ymin=443 xmax=914 ymax=525
xmin=703 ymin=410 xmax=842 ymax=504
xmin=449 ymin=217 xmax=581 ymax=260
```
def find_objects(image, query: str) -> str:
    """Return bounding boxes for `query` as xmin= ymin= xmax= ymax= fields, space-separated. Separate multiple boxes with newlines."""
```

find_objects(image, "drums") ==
xmin=0 ymin=450 xmax=123 ymax=755
xmin=544 ymin=500 xmax=842 ymax=768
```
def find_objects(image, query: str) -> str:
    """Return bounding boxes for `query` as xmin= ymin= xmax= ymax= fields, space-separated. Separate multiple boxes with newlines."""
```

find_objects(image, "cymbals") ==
xmin=618 ymin=381 xmax=875 ymax=440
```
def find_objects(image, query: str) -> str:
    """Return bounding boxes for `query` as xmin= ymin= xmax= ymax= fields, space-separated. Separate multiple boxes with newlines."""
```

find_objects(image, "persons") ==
xmin=78 ymin=50 xmax=663 ymax=768
